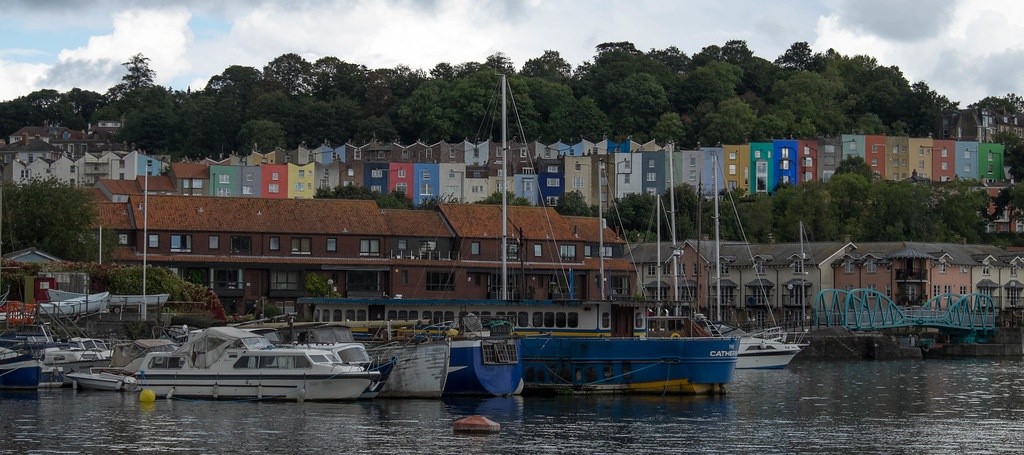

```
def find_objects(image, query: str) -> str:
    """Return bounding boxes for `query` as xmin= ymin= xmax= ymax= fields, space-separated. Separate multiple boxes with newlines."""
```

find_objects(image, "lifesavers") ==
xmin=670 ymin=333 xmax=680 ymax=337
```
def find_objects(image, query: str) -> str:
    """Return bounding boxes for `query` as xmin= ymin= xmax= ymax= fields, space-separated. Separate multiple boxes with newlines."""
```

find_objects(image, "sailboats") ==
xmin=0 ymin=73 xmax=804 ymax=402
